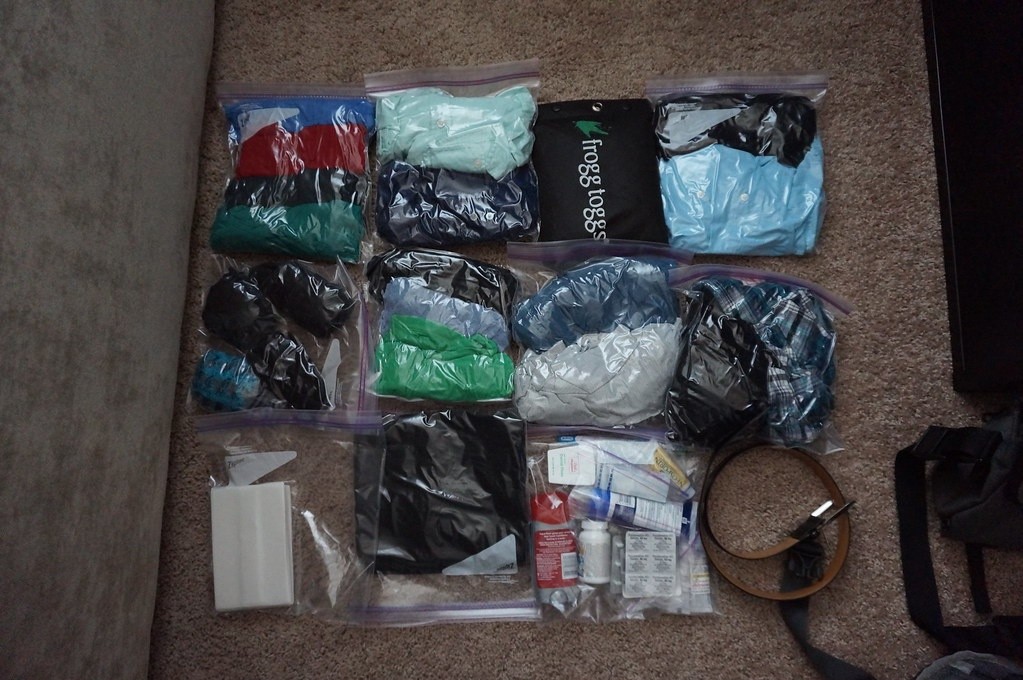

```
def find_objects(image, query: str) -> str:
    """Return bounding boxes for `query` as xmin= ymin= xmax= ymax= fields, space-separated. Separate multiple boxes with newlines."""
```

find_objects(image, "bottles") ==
xmin=533 ymin=494 xmax=579 ymax=604
xmin=578 ymin=520 xmax=612 ymax=584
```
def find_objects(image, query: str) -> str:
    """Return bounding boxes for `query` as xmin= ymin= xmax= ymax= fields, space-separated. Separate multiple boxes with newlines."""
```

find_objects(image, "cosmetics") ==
xmin=587 ymin=487 xmax=684 ymax=535
xmin=531 ymin=491 xmax=578 ymax=605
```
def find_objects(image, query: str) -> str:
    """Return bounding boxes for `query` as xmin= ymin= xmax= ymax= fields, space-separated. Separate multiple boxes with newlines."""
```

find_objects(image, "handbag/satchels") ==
xmin=929 ymin=405 xmax=1023 ymax=552
